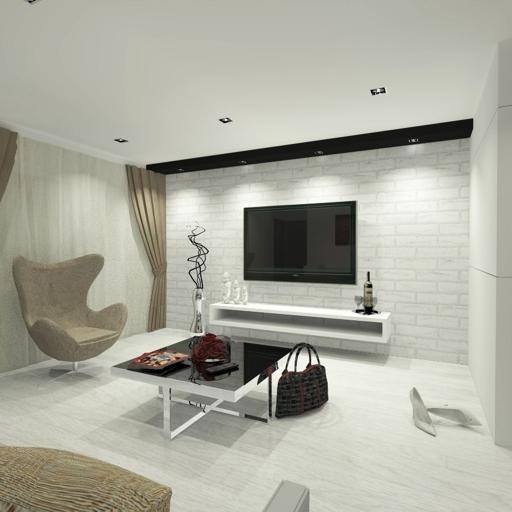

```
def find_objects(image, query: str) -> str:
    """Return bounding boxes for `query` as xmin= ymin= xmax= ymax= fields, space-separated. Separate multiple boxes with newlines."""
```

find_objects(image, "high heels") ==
xmin=409 ymin=387 xmax=436 ymax=437
xmin=426 ymin=404 xmax=481 ymax=426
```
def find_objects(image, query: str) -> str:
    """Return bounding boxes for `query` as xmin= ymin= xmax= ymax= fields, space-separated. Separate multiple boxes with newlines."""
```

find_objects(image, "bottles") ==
xmin=222 ymin=271 xmax=248 ymax=305
xmin=364 ymin=271 xmax=373 ymax=313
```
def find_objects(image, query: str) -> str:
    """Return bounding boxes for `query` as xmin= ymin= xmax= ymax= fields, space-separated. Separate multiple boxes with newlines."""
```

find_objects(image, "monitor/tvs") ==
xmin=243 ymin=200 xmax=357 ymax=285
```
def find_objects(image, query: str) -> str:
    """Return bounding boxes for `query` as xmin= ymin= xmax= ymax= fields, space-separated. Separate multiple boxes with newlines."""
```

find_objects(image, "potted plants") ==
xmin=187 ymin=221 xmax=209 ymax=333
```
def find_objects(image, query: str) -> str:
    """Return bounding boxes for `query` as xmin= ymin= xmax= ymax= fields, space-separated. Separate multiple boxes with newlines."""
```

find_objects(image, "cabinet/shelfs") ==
xmin=209 ymin=300 xmax=392 ymax=344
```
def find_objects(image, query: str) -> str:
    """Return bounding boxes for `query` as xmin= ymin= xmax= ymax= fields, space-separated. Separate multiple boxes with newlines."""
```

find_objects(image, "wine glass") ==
xmin=352 ymin=296 xmax=377 ymax=313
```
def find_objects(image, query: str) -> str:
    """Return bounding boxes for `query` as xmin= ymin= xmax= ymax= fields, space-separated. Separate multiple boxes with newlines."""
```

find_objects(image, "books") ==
xmin=133 ymin=346 xmax=190 ymax=370
xmin=126 ymin=351 xmax=152 ymax=370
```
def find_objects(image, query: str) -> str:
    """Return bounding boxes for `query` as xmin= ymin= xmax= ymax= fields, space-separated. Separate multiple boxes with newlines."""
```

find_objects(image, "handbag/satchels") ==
xmin=275 ymin=342 xmax=329 ymax=419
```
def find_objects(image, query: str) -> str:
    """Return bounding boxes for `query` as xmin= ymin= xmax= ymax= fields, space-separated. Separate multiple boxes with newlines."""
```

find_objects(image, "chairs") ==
xmin=12 ymin=253 xmax=128 ymax=370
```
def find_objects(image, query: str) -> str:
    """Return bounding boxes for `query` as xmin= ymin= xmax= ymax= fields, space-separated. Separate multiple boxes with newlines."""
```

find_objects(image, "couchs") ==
xmin=0 ymin=445 xmax=172 ymax=511
